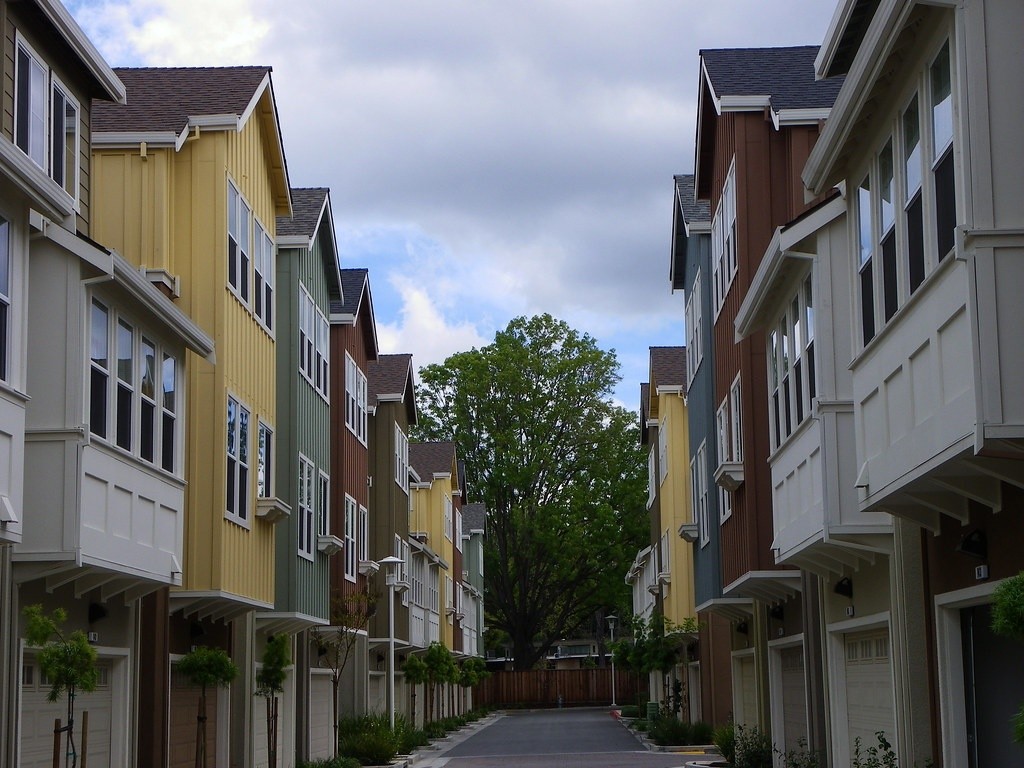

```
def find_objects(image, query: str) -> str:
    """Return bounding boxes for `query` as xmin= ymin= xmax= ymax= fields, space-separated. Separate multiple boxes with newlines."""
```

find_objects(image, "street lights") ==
xmin=604 ymin=615 xmax=619 ymax=706
xmin=373 ymin=555 xmax=406 ymax=740
